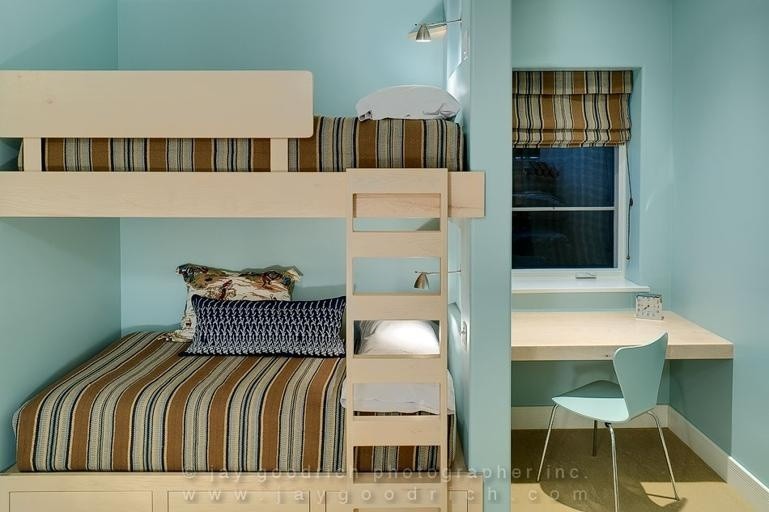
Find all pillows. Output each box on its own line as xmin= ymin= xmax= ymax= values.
xmin=335 ymin=314 xmax=456 ymax=418
xmin=156 ymin=256 xmax=345 ymax=359
xmin=354 ymin=82 xmax=462 ymax=122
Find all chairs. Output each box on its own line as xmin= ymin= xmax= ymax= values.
xmin=535 ymin=330 xmax=682 ymax=512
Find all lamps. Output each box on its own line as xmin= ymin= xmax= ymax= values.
xmin=413 ymin=16 xmax=462 ymax=43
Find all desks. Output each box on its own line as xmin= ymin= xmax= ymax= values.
xmin=509 ymin=307 xmax=733 ymax=363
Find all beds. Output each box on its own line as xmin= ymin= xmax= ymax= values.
xmin=0 ymin=68 xmax=486 ymax=512
